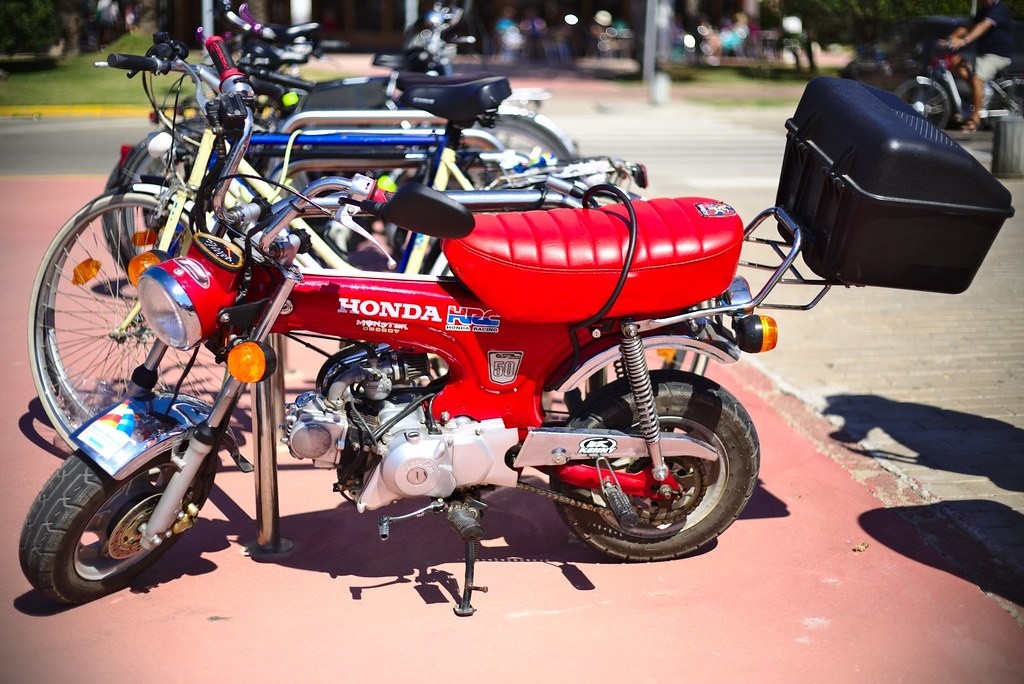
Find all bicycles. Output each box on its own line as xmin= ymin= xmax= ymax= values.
xmin=896 ymin=38 xmax=1024 ymax=138
xmin=27 ymin=0 xmax=650 ymax=452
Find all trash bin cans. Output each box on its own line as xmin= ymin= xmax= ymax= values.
xmin=991 ymin=114 xmax=1023 ymax=182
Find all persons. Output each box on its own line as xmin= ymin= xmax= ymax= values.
xmin=856 ymin=42 xmax=885 ymax=63
xmin=82 ymin=0 xmax=137 ymax=45
xmin=939 ymin=0 xmax=1015 ymax=130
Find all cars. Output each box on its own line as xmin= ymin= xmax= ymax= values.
xmin=842 ymin=14 xmax=1017 ymax=122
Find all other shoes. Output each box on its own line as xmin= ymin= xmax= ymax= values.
xmin=962 ymin=120 xmax=979 ymax=132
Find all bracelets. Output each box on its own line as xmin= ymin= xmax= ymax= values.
xmin=962 ymin=37 xmax=969 ymax=44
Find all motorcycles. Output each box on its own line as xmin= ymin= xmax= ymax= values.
xmin=19 ymin=34 xmax=1016 ymax=618
